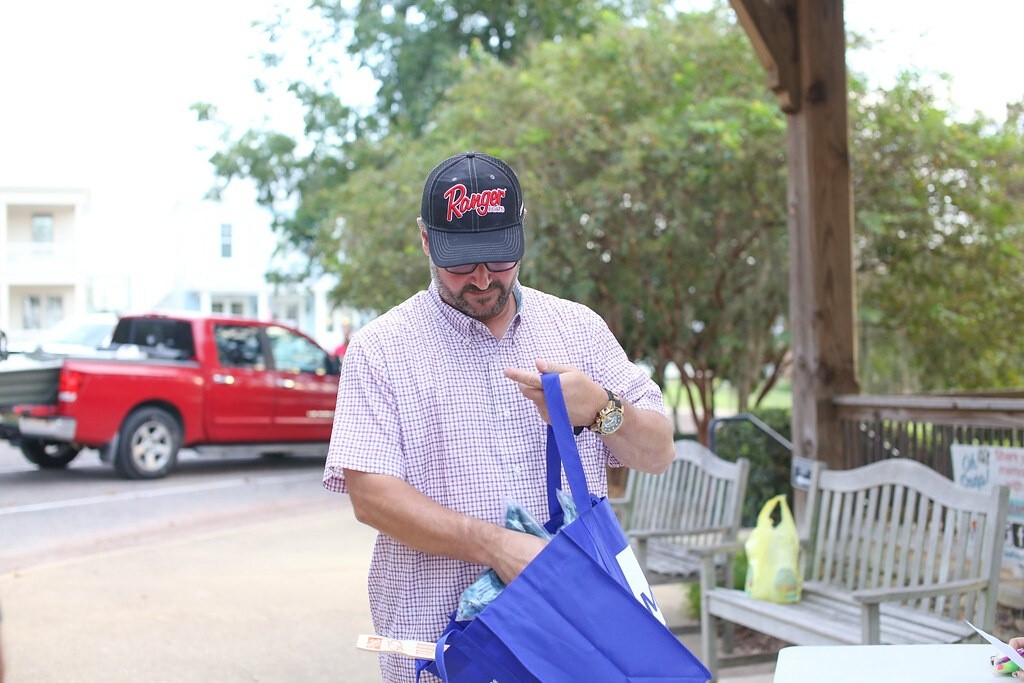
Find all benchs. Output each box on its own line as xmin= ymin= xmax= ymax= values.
xmin=676 ymin=455 xmax=1011 ymax=683
xmin=609 ymin=440 xmax=751 ymax=653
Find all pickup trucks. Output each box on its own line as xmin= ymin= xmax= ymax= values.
xmin=0 ymin=314 xmax=348 ymax=479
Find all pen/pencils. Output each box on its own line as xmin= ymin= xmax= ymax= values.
xmin=992 ymin=648 xmax=1023 ymax=674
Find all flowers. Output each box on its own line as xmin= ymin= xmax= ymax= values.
xmin=990 ymin=648 xmax=1024 ymax=679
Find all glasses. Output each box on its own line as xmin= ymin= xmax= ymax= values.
xmin=445 ymin=261 xmax=517 ymax=274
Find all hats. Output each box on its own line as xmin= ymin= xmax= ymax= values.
xmin=420 ymin=152 xmax=524 ymax=267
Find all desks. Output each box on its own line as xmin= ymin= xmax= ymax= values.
xmin=773 ymin=644 xmax=1024 ymax=683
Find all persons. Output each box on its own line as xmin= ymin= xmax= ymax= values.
xmin=321 ymin=151 xmax=676 ymax=683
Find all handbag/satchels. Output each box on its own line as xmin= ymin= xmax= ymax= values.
xmin=744 ymin=494 xmax=802 ymax=603
xmin=415 ymin=374 xmax=711 ymax=683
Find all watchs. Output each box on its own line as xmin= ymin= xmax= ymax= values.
xmin=573 ymin=388 xmax=625 ymax=437
